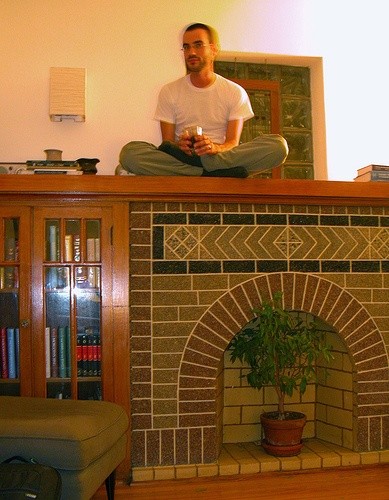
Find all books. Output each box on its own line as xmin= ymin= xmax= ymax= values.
xmin=353 ymin=165 xmax=389 ymax=182
xmin=45 ymin=327 xmax=102 ymax=378
xmin=48 ymin=225 xmax=101 ymax=289
xmin=0 ymin=327 xmax=20 ymax=379
xmin=0 ymin=236 xmax=19 ymax=289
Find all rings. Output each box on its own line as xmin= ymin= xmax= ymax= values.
xmin=208 ymin=145 xmax=212 ymax=149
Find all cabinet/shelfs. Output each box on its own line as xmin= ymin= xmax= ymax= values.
xmin=0 ymin=200 xmax=133 ymax=480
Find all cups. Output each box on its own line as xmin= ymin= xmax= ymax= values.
xmin=76 ymin=158 xmax=100 ymax=175
xmin=45 ymin=149 xmax=62 ymax=161
xmin=185 ymin=126 xmax=202 ymax=153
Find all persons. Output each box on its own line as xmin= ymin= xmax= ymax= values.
xmin=119 ymin=23 xmax=289 ymax=178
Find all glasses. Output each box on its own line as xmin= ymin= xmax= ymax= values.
xmin=180 ymin=43 xmax=216 ymax=51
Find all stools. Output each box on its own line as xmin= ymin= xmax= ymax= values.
xmin=0 ymin=396 xmax=131 ymax=500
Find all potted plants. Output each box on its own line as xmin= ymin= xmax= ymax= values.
xmin=227 ymin=291 xmax=336 ymax=457
